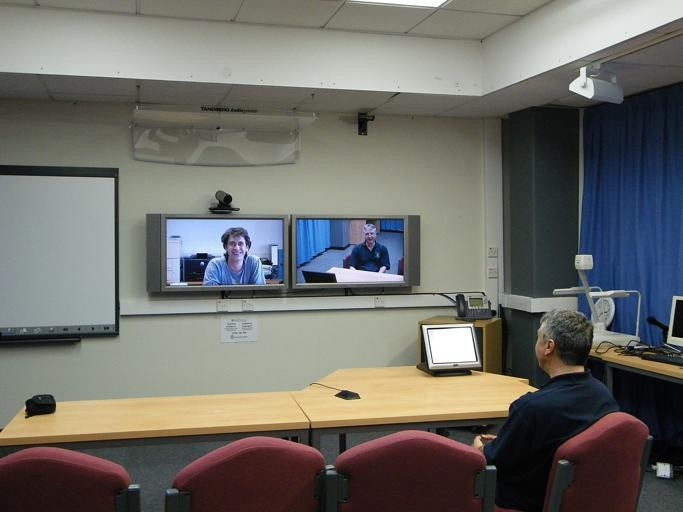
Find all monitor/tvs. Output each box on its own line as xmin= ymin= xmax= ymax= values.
xmin=146 ymin=212 xmax=290 ymax=293
xmin=417 ymin=322 xmax=483 ymax=378
xmin=667 ymin=295 xmax=683 ymax=347
xmin=291 ymin=213 xmax=421 ymax=290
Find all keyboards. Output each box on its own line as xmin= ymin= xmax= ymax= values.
xmin=643 ymin=354 xmax=683 ymax=367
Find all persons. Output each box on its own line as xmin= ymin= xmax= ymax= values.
xmin=203 ymin=227 xmax=267 ymax=285
xmin=472 ymin=308 xmax=621 ymax=511
xmin=346 ymin=224 xmax=390 ymax=273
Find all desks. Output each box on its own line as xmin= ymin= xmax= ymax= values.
xmin=589 ymin=334 xmax=683 ymax=471
xmin=0 ymin=392 xmax=312 ymax=447
xmin=292 ymin=366 xmax=539 ymax=457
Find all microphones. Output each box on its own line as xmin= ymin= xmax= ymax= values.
xmin=646 ymin=315 xmax=668 ymax=331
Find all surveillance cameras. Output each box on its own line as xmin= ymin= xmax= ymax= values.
xmin=209 ymin=190 xmax=241 ymax=217
xmin=356 ymin=110 xmax=376 ymax=137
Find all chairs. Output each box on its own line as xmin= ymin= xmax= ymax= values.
xmin=540 ymin=413 xmax=653 ymax=511
xmin=164 ymin=436 xmax=333 ymax=512
xmin=0 ymin=444 xmax=140 ymax=512
xmin=333 ymin=430 xmax=498 ymax=512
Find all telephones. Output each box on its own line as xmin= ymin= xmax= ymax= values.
xmin=454 ymin=289 xmax=494 ymax=321
